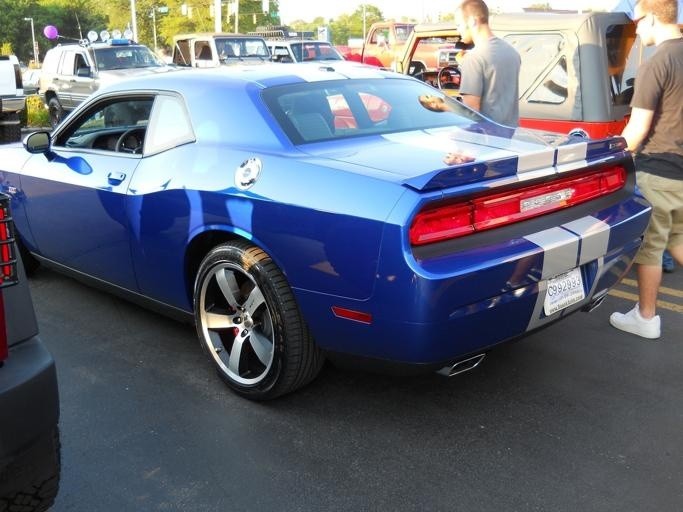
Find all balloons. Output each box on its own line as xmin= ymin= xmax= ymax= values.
xmin=42 ymin=25 xmax=58 ymax=41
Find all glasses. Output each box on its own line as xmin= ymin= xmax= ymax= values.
xmin=631 ymin=14 xmax=649 ymax=29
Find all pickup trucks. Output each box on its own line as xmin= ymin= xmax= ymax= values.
xmin=341 ymin=19 xmax=412 ymax=63
xmin=0 ymin=56 xmax=26 ymax=144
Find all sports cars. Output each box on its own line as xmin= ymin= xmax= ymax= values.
xmin=0 ymin=61 xmax=652 ymax=398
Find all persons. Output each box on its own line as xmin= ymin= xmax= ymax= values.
xmin=608 ymin=0 xmax=683 ymax=338
xmin=455 ymin=1 xmax=522 ymax=129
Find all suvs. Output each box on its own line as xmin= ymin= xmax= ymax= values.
xmin=37 ymin=40 xmax=185 ymax=130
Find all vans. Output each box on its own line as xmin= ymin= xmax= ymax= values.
xmin=382 ymin=12 xmax=636 ymax=126
xmin=264 ymin=41 xmax=345 ymax=63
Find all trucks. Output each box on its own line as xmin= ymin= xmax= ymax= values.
xmin=172 ymin=34 xmax=271 ymax=71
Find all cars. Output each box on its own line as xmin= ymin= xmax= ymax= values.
xmin=0 ymin=199 xmax=60 ymax=512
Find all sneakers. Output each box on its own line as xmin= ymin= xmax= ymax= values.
xmin=609 ymin=301 xmax=661 ymax=339
